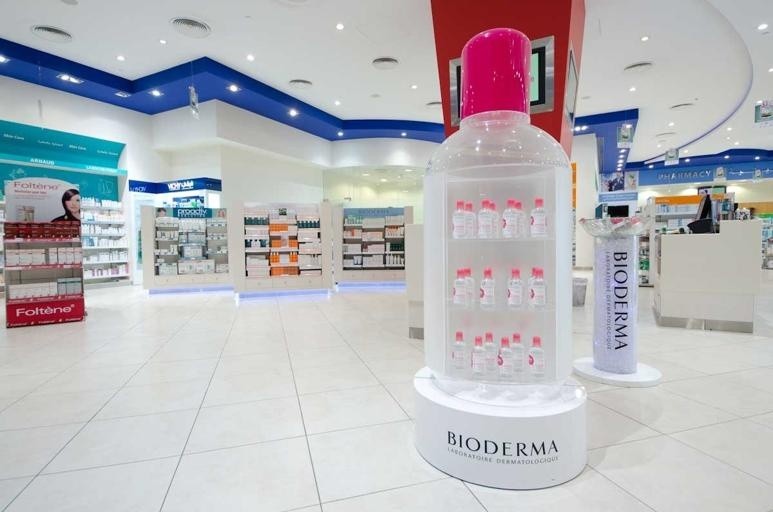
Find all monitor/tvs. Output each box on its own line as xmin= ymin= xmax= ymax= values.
xmin=695 ymin=195 xmax=712 ymax=220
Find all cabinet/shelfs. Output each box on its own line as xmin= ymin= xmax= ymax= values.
xmin=1 ymin=152 xmax=130 ymax=299
xmin=635 ymin=193 xmax=773 ymax=287
xmin=4 ymin=221 xmax=85 ymax=328
xmin=423 ymin=109 xmax=573 ymax=409
xmin=141 ymin=203 xmax=413 ymax=294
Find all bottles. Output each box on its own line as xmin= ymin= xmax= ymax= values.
xmin=736 ymin=207 xmax=751 ymax=220
xmin=289 ymin=251 xmax=298 ymax=263
xmin=389 ymin=223 xmax=402 ymax=251
xmin=452 ymin=328 xmax=545 ymax=381
xmin=579 ymin=216 xmax=646 ymax=237
xmin=81 ymin=224 xmax=125 ymax=236
xmin=270 ymin=252 xmax=279 ymax=263
xmin=246 ymin=216 xmax=267 ymax=224
xmin=81 ymin=196 xmax=123 ymax=209
xmin=452 ymin=199 xmax=546 ymax=239
xmin=455 ymin=264 xmax=546 ymax=312
xmin=97 ymin=213 xmax=123 ymax=222
xmin=299 ymin=219 xmax=320 ymax=229
xmin=722 ymin=198 xmax=731 ymax=211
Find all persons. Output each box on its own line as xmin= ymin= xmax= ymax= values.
xmin=157 ymin=208 xmax=166 ymax=217
xmin=49 ymin=188 xmax=80 ymax=221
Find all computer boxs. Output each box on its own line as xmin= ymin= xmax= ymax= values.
xmin=687 ymin=219 xmax=714 ymax=233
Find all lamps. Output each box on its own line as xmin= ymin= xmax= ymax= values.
xmin=169 ymin=16 xmax=211 ymax=121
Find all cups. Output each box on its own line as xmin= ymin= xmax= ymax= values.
xmin=722 ymin=213 xmax=727 ymax=221
